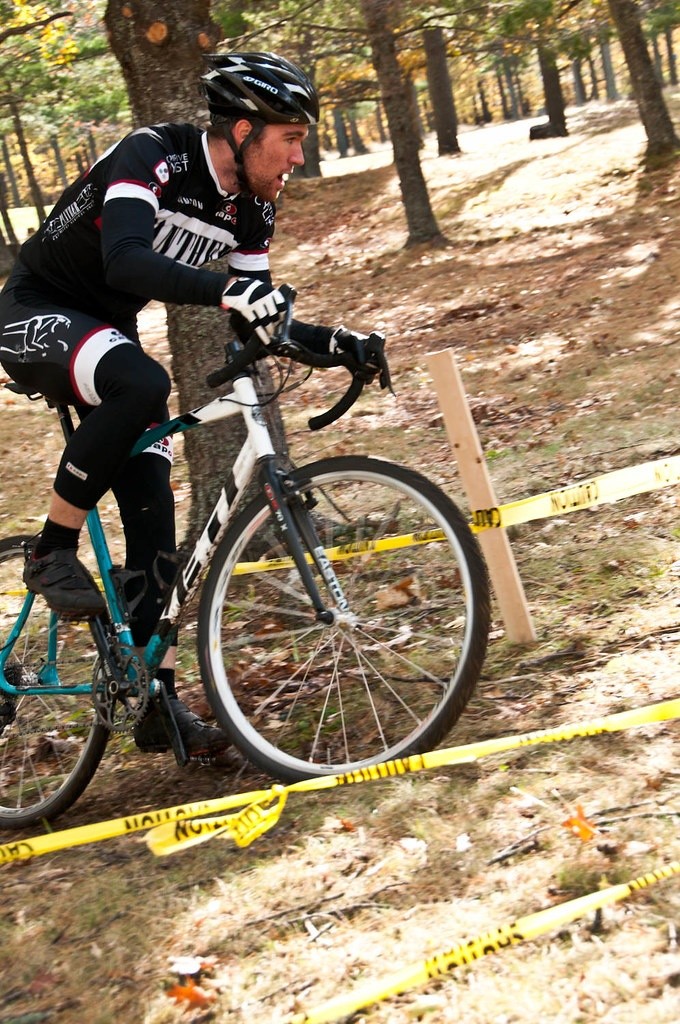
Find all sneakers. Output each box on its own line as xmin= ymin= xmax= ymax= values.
xmin=134 ymin=699 xmax=233 ymax=756
xmin=23 ymin=547 xmax=106 ymax=618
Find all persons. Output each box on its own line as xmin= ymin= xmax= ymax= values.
xmin=0 ymin=49 xmax=383 ymax=752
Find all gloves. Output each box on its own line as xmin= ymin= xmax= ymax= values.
xmin=329 ymin=324 xmax=391 ymax=390
xmin=218 ymin=277 xmax=288 ymax=344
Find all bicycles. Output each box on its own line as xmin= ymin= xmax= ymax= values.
xmin=0 ymin=282 xmax=490 ymax=831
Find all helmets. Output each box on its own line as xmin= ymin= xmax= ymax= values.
xmin=200 ymin=51 xmax=318 ymax=124
